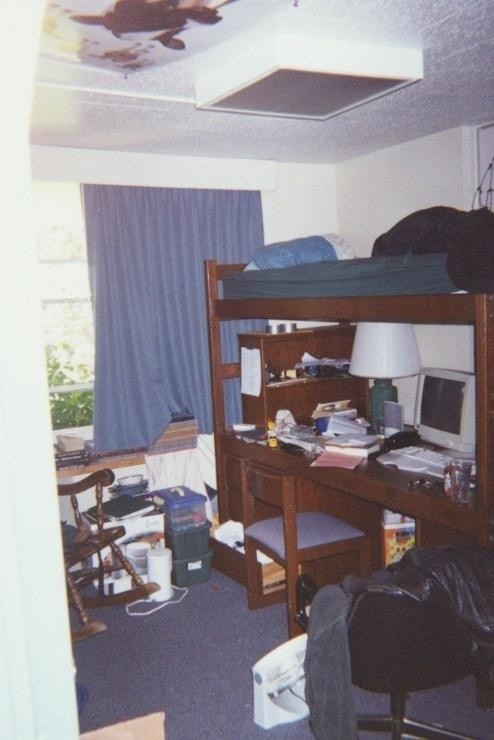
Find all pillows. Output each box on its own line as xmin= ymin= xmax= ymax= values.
xmin=250 ymin=233 xmax=355 ymax=269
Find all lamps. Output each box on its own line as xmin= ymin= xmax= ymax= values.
xmin=347 ymin=322 xmax=423 ymax=429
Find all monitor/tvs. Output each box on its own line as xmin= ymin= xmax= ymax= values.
xmin=413 ymin=367 xmax=476 ymax=459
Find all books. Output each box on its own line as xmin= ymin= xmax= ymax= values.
xmin=311 ymin=399 xmax=380 ymax=458
xmin=52 ymin=410 xmax=198 ymax=477
xmin=87 ymin=495 xmax=155 ymax=523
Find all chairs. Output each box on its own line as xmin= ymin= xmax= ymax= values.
xmin=297 ymin=545 xmax=493 ymax=740
xmin=57 ymin=468 xmax=160 ymax=642
xmin=236 ymin=456 xmax=372 ymax=640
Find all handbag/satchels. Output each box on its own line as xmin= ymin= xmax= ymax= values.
xmin=339 ymin=557 xmax=480 ymax=692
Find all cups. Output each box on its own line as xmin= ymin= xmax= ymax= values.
xmin=450 ymin=461 xmax=472 ymax=504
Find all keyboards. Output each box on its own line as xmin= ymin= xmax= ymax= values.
xmin=377 ymin=445 xmax=476 ymax=478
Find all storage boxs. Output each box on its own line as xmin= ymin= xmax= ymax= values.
xmin=78 ymin=486 xmax=212 ymax=594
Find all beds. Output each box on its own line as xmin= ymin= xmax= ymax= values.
xmin=203 ymin=253 xmax=494 ymax=325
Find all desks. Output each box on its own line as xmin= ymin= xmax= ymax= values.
xmin=226 ymin=325 xmax=476 ymax=588
